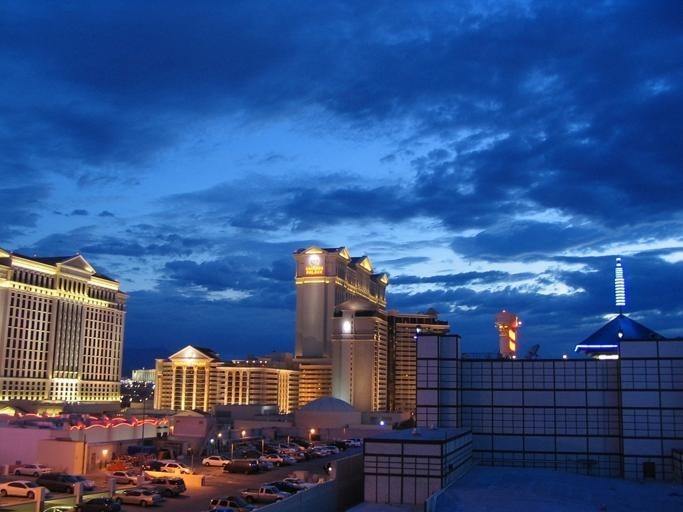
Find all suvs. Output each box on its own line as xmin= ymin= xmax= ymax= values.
xmin=142 ymin=476 xmax=186 ymax=496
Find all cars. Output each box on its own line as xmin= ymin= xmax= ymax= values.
xmin=76 ymin=498 xmax=121 ymax=512
xmin=0 ymin=480 xmax=50 ymax=499
xmin=112 ymin=488 xmax=161 ymax=507
xmin=112 ymin=470 xmax=138 ymax=484
xmin=140 ymin=485 xmax=160 ymax=493
xmin=13 ymin=463 xmax=52 ymax=477
xmin=200 ymin=438 xmax=363 ymax=475
xmin=35 ymin=472 xmax=95 ymax=494
xmin=159 ymin=463 xmax=191 ymax=475
xmin=42 ymin=505 xmax=75 ymax=512
xmin=208 ymin=477 xmax=316 ymax=511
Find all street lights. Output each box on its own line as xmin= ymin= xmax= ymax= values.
xmin=133 ymin=380 xmax=156 ymax=440
xmin=309 ymin=429 xmax=315 ymax=441
